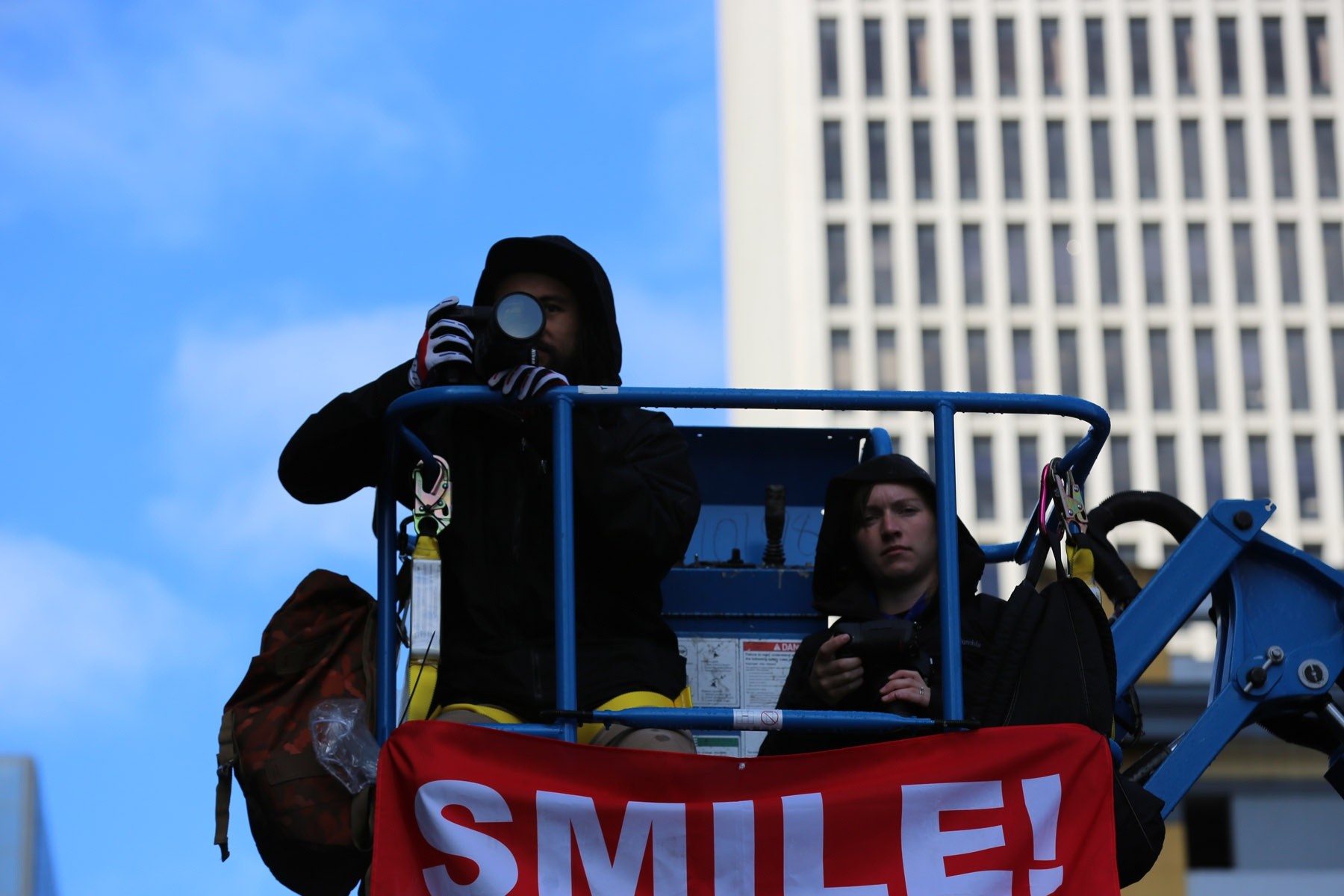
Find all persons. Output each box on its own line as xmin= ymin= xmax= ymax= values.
xmin=275 ymin=236 xmax=702 ymax=761
xmin=757 ymin=453 xmax=1033 ymax=761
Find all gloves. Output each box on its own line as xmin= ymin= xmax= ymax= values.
xmin=488 ymin=362 xmax=572 ymax=406
xmin=409 ymin=296 xmax=476 ymax=389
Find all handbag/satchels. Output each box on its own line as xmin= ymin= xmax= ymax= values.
xmin=1007 ymin=529 xmax=1165 ymax=889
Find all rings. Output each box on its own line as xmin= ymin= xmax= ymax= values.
xmin=919 ymin=686 xmax=924 ymax=695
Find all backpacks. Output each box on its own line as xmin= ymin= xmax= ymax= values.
xmin=214 ymin=569 xmax=410 ymax=896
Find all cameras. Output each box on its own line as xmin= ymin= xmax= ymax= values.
xmin=444 ymin=290 xmax=545 ymax=375
xmin=830 ymin=617 xmax=935 ymax=688
